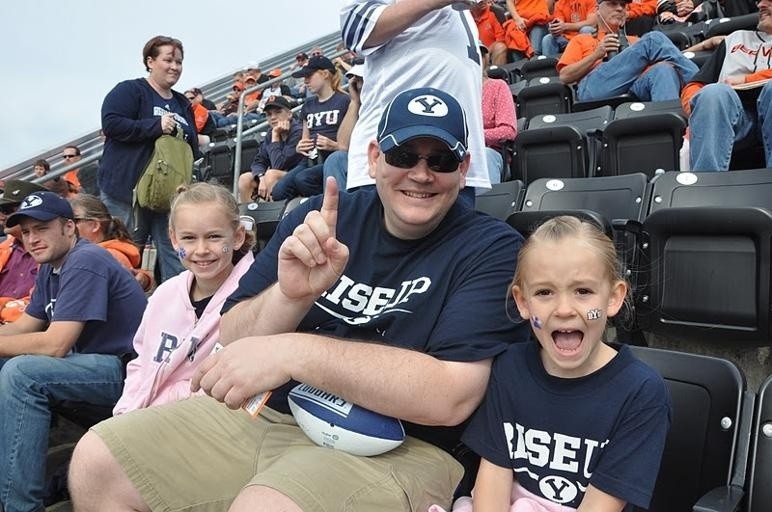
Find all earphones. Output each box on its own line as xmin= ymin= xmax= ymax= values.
xmin=596 ymin=9 xmax=600 ymax=15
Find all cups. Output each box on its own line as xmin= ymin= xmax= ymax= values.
xmin=451 ymin=2 xmax=467 ymax=12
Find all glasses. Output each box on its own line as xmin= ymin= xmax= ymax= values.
xmin=384 ymin=149 xmax=460 ymax=173
xmin=184 ymin=96 xmax=199 ymax=101
xmin=61 ymin=153 xmax=81 ymax=160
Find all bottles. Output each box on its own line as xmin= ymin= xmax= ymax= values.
xmin=680 ymin=136 xmax=689 ymax=171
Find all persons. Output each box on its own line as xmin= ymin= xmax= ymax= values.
xmin=680 ymin=0 xmax=772 ymax=172
xmin=450 ymin=215 xmax=673 ymax=512
xmin=182 ymin=42 xmax=364 ymax=204
xmin=0 ymin=177 xmax=153 ymax=363
xmin=473 ymin=0 xmax=727 ymax=183
xmin=109 ymin=183 xmax=256 ymax=417
xmin=70 ymin=87 xmax=525 ymax=512
xmin=35 ymin=145 xmax=101 ymax=197
xmin=339 ymin=0 xmax=491 ymax=206
xmin=0 ymin=190 xmax=148 ymax=512
xmin=97 ymin=36 xmax=200 ymax=284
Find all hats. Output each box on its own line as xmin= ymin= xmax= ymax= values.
xmin=232 ymin=50 xmax=368 ymax=114
xmin=1 ymin=178 xmax=80 ymax=230
xmin=376 ymin=86 xmax=469 ymax=165
xmin=472 ymin=40 xmax=493 ymax=57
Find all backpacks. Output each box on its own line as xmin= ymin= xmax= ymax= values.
xmin=131 ymin=126 xmax=195 ymax=213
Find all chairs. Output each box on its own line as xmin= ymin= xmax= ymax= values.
xmin=21 ymin=1 xmax=772 ymax=512
